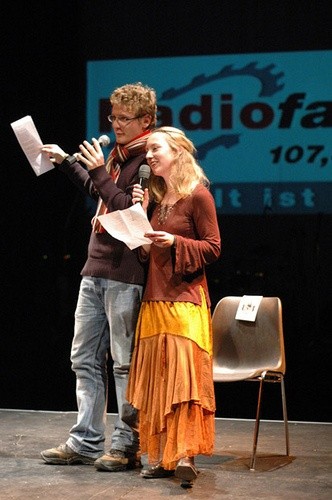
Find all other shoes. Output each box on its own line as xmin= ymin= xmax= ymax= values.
xmin=140 ymin=464 xmax=176 ymax=478
xmin=175 ymin=460 xmax=198 ymax=480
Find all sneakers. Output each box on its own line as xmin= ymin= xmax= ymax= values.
xmin=94 ymin=448 xmax=137 ymax=471
xmin=40 ymin=443 xmax=98 ymax=464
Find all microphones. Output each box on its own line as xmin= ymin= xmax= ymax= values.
xmin=66 ymin=135 xmax=110 ymax=166
xmin=136 ymin=165 xmax=151 ymax=206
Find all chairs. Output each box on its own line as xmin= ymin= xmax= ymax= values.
xmin=212 ymin=296 xmax=293 ymax=469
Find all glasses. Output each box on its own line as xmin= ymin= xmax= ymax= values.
xmin=108 ymin=113 xmax=146 ymax=123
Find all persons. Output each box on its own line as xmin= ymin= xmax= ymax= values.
xmin=126 ymin=127 xmax=221 ymax=488
xmin=40 ymin=82 xmax=155 ymax=470
